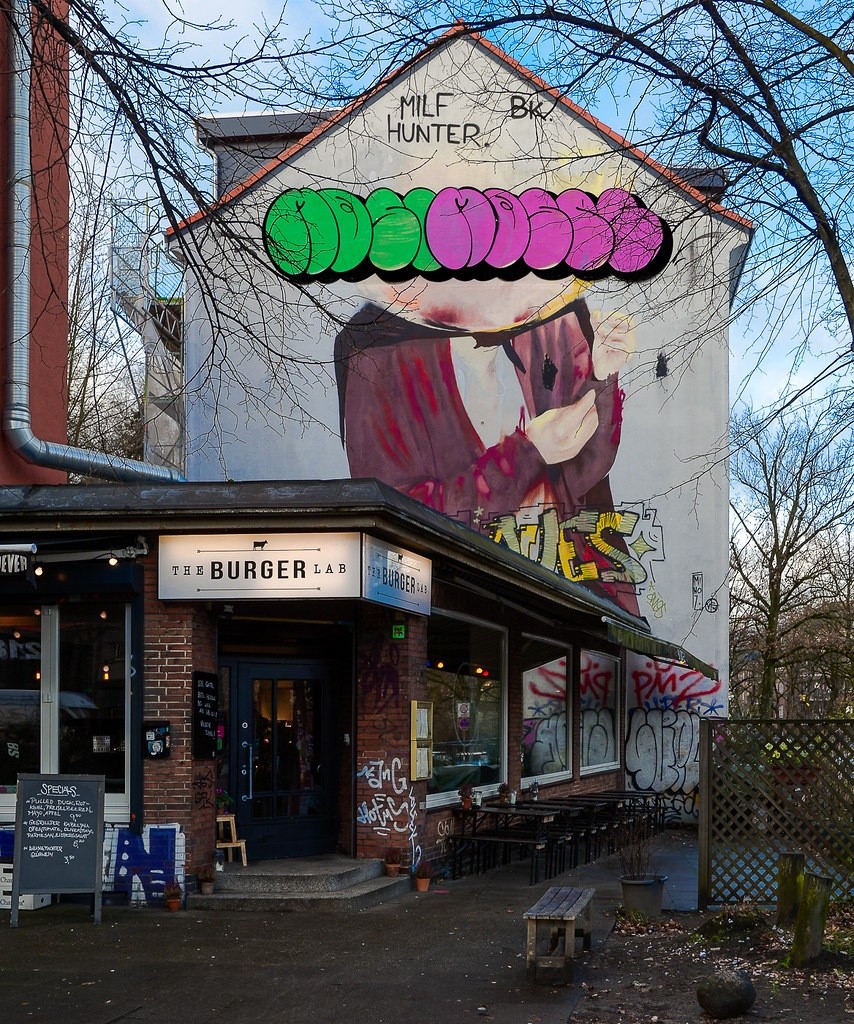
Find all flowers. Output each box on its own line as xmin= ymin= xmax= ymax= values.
xmin=458 ymin=783 xmax=476 ymax=800
xmin=528 ymin=779 xmax=543 ymax=797
xmin=497 ymin=781 xmax=516 ymax=795
xmin=214 ymin=850 xmax=224 ymax=866
xmin=162 ymin=875 xmax=184 ymax=900
xmin=215 ymin=786 xmax=235 ymax=808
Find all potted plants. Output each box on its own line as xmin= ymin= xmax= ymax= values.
xmin=604 ymin=798 xmax=669 ymax=925
xmin=414 ymin=859 xmax=437 ymax=891
xmin=383 ymin=847 xmax=400 ymax=877
xmin=194 ymin=865 xmax=215 ymax=894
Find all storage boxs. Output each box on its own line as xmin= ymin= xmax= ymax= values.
xmin=0 ymin=863 xmax=13 ymax=886
xmin=0 ymin=886 xmax=51 ymax=910
xmin=0 ymin=825 xmax=14 ymax=863
xmin=0 ymin=794 xmax=18 ymax=822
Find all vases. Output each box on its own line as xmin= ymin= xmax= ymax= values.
xmin=461 ymin=797 xmax=473 ymax=809
xmin=500 ymin=793 xmax=510 ymax=803
xmin=533 ymin=788 xmax=538 ymax=801
xmin=216 ymin=809 xmax=225 ymax=815
xmin=215 ymin=861 xmax=225 ymax=872
xmin=167 ymin=899 xmax=180 ymax=912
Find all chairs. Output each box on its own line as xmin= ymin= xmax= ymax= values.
xmin=215 ymin=815 xmax=247 ymax=865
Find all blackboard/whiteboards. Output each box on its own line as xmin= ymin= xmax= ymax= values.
xmin=192 ymin=671 xmax=219 ymax=761
xmin=13 ymin=773 xmax=105 ymax=893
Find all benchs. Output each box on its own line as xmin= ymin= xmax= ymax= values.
xmin=448 ymin=806 xmax=671 ymax=887
xmin=522 ymin=887 xmax=596 ymax=967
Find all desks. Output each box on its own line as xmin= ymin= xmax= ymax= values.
xmin=451 ymin=790 xmax=664 ymax=884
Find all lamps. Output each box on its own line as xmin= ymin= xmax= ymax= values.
xmin=35 ymin=558 xmax=44 ymax=576
xmin=87 ymin=552 xmax=120 ymax=566
xmin=475 ymin=662 xmax=482 ymax=675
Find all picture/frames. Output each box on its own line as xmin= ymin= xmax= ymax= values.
xmin=115 ymin=641 xmax=125 ymax=661
